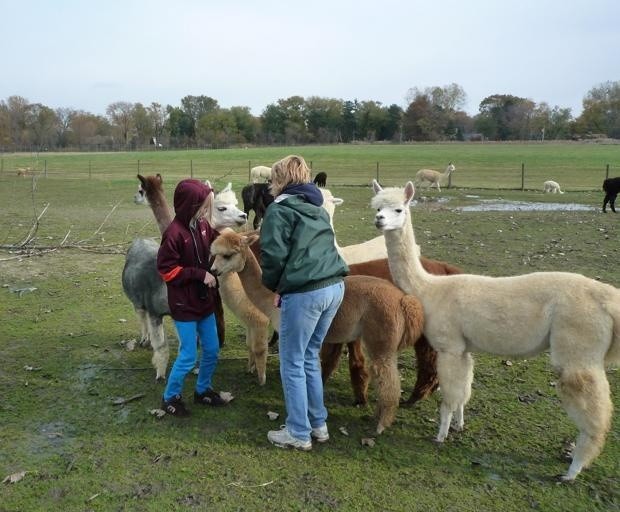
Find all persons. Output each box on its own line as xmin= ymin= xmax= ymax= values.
xmin=158 ymin=180 xmax=227 ymax=417
xmin=258 ymin=154 xmax=350 ymax=450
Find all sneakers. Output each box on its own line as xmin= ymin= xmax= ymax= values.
xmin=311 ymin=423 xmax=330 ymax=444
xmin=267 ymin=426 xmax=313 ymax=452
xmin=194 ymin=387 xmax=226 ymax=407
xmin=161 ymin=394 xmax=192 ymax=418
